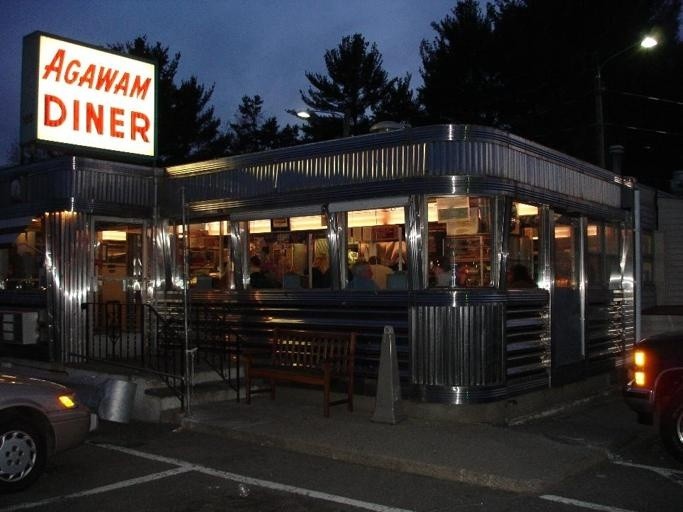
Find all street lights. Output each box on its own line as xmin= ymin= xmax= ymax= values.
xmin=595 ymin=34 xmax=659 ymax=168
xmin=298 ymin=108 xmax=354 ymax=140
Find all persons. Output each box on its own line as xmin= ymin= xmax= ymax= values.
xmin=154 ymin=245 xmax=579 ymax=293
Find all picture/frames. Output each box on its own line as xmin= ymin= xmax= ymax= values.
xmin=271 ymin=217 xmax=289 ymax=231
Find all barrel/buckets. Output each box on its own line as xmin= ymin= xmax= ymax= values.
xmin=98 ymin=379 xmax=137 ymax=424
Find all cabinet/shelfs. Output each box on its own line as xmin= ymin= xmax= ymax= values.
xmin=442 ymin=235 xmax=534 ymax=287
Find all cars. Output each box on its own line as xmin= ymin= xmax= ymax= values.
xmin=626 ymin=332 xmax=683 ymax=457
xmin=0 ymin=374 xmax=90 ymax=491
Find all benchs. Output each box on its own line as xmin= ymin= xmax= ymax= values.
xmin=245 ymin=329 xmax=356 ymax=417
